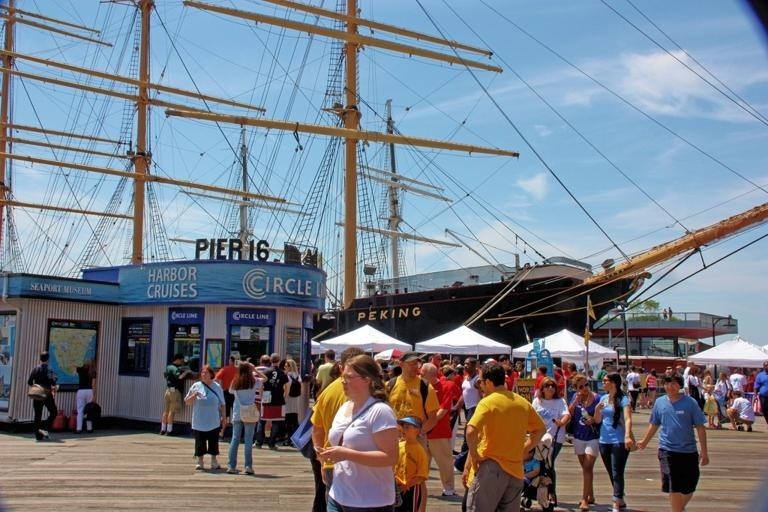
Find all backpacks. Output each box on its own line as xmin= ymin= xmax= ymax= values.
xmin=289 ymin=375 xmax=301 ymax=397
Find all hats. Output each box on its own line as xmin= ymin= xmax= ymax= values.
xmin=396 ymin=417 xmax=421 ymax=429
xmin=400 ymin=352 xmax=426 ymax=363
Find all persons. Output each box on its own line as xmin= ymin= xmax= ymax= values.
xmin=308 ymin=350 xmax=710 ymax=511
xmin=70 ymin=357 xmax=102 ymax=433
xmin=25 ymin=349 xmax=57 ymax=441
xmin=184 ymin=353 xmax=302 ymax=474
xmin=685 ymin=360 xmax=768 ymax=431
xmin=160 ymin=353 xmax=193 ymax=436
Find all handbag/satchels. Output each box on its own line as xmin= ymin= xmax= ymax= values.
xmin=633 ymin=382 xmax=641 ymax=388
xmin=290 ymin=407 xmax=321 ymax=458
xmin=261 ymin=390 xmax=272 ymax=404
xmin=28 ymin=383 xmax=48 ymax=401
xmin=239 ymin=403 xmax=261 ymax=423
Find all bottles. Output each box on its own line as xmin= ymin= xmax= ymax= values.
xmin=580 ymin=408 xmax=589 ymax=419
xmin=191 ymin=327 xmax=199 ymax=333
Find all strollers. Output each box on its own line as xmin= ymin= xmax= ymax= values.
xmin=521 ymin=420 xmax=561 ymax=512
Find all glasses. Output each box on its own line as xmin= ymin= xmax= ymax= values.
xmin=602 ymin=378 xmax=609 ymax=384
xmin=579 ymin=383 xmax=589 ymax=389
xmin=543 ymin=383 xmax=555 ymax=388
xmin=341 ymin=373 xmax=360 ymax=382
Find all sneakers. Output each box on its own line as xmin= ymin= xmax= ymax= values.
xmin=211 ymin=460 xmax=220 ymax=469
xmin=196 ymin=461 xmax=204 ymax=470
xmin=165 ymin=431 xmax=176 ymax=435
xmin=38 ymin=429 xmax=50 ymax=438
xmin=159 ymin=430 xmax=166 ymax=435
xmin=442 ymin=489 xmax=464 ymax=502
xmin=245 ymin=466 xmax=254 ymax=473
xmin=707 ymin=423 xmax=752 ymax=432
xmin=226 ymin=467 xmax=239 ymax=473
xmin=252 ymin=443 xmax=261 ymax=449
xmin=607 ymin=504 xmax=627 ymax=510
